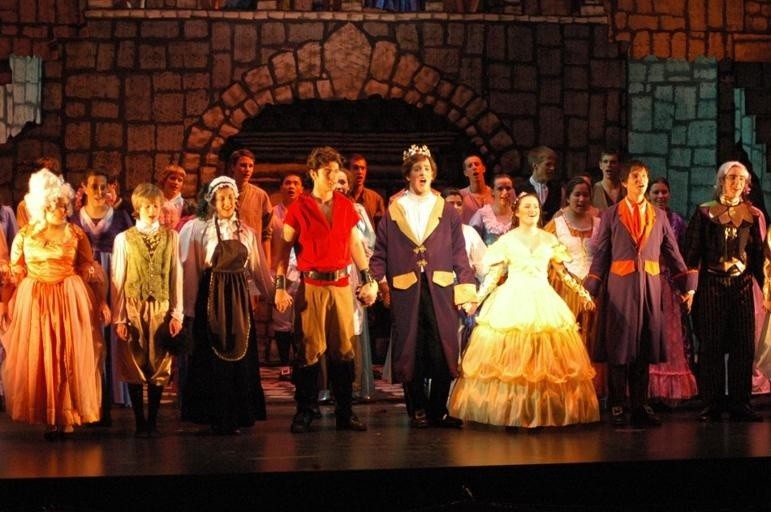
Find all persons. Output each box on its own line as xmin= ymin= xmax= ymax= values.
xmin=3 ymin=169 xmax=111 ymax=435
xmin=178 ymin=176 xmax=295 ymax=431
xmin=20 ymin=143 xmax=770 ymax=410
xmin=356 ymin=143 xmax=477 ymax=427
xmin=577 ymin=156 xmax=698 ymax=430
xmin=110 ymin=182 xmax=183 ymax=439
xmin=442 ymin=191 xmax=599 ymax=429
xmin=682 ymin=159 xmax=767 ymax=425
xmin=272 ymin=146 xmax=379 ymax=431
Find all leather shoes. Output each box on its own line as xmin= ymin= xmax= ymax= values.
xmin=608 ymin=404 xmax=658 ymax=425
xmin=410 ymin=407 xmax=463 ymax=427
xmin=698 ymin=399 xmax=764 ymax=422
xmin=291 ymin=409 xmax=366 ymax=433
xmin=45 ymin=424 xmax=75 ymax=440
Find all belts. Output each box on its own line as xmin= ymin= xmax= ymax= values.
xmin=300 ymin=269 xmax=347 ymax=280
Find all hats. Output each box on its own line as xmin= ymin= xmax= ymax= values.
xmin=207 ymin=176 xmax=239 ymax=199
